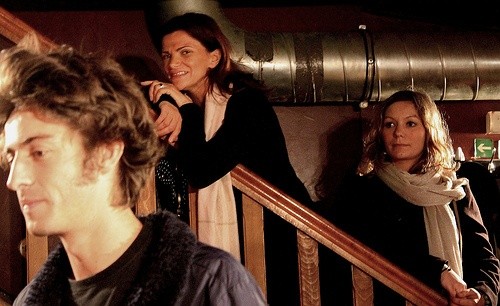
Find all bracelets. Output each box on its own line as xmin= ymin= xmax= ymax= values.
xmin=442 ymin=266 xmax=452 ymax=273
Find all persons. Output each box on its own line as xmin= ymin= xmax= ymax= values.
xmin=0 ymin=46 xmax=267 ymax=306
xmin=317 ymin=90 xmax=500 ymax=306
xmin=117 ymin=11 xmax=328 ymax=305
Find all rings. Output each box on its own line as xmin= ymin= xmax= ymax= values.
xmin=158 ymin=83 xmax=165 ymax=89
